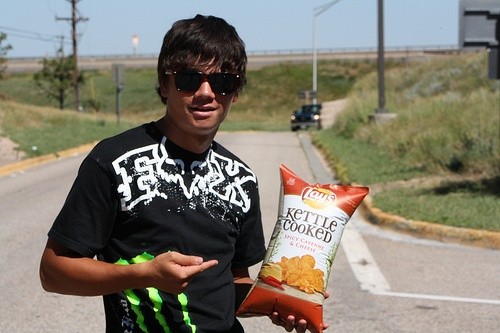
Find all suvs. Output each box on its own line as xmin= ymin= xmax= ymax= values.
xmin=290 ymin=103 xmax=323 ymax=131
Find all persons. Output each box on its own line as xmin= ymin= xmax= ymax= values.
xmin=39 ymin=14 xmax=329 ymax=333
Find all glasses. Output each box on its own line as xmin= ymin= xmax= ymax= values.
xmin=165 ymin=67 xmax=241 ymax=96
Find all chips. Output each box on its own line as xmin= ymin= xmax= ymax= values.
xmin=281 ymin=256 xmax=325 ymax=294
